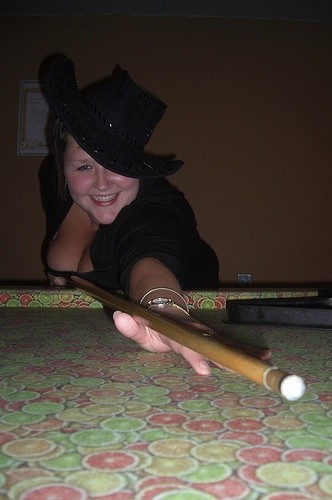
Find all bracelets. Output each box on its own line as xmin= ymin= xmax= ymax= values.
xmin=137 ymin=286 xmax=190 ymax=313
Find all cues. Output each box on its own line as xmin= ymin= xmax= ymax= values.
xmin=66 ymin=276 xmax=305 ymax=403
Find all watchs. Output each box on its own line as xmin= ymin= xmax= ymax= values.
xmin=143 ymin=296 xmax=190 ymax=317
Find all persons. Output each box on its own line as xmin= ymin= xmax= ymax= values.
xmin=44 ymin=65 xmax=274 ymax=377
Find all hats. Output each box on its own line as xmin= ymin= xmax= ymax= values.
xmin=38 ymin=52 xmax=177 ymax=178
